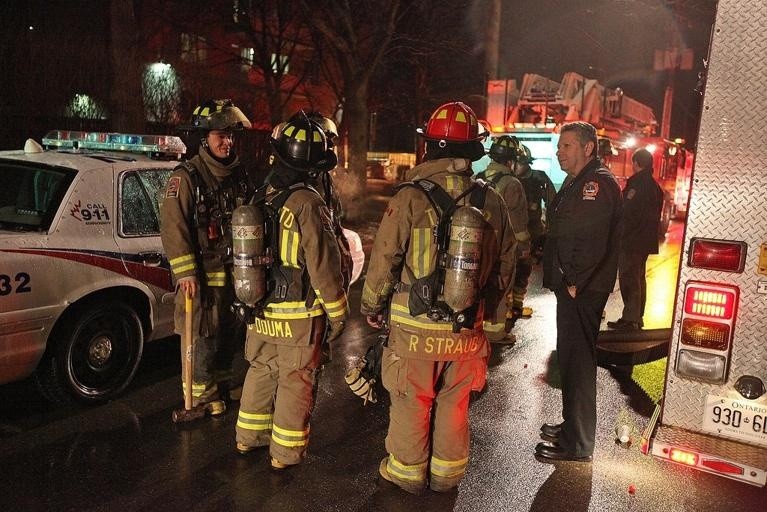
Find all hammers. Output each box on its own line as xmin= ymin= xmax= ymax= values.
xmin=172 ymin=290 xmax=204 ymax=422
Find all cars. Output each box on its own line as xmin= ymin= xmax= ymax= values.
xmin=0 ymin=128 xmax=367 ymax=408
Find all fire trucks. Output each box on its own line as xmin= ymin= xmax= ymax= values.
xmin=634 ymin=1 xmax=767 ymax=490
xmin=473 ymin=73 xmax=686 ymax=239
xmin=671 ymin=148 xmax=693 ymax=221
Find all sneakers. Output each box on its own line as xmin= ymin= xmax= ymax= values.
xmin=203 ymin=398 xmax=229 ymax=414
xmin=237 ymin=442 xmax=288 ymax=469
xmin=522 ymin=307 xmax=533 ymax=317
xmin=379 ymin=456 xmax=393 ymax=481
xmin=489 ymin=334 xmax=516 ymax=345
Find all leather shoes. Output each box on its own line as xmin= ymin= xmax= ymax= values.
xmin=606 ymin=321 xmax=637 ymax=328
xmin=536 ymin=443 xmax=592 ymax=460
xmin=541 ymin=423 xmax=565 ymax=437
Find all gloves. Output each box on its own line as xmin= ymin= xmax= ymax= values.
xmin=326 ymin=323 xmax=345 ymax=345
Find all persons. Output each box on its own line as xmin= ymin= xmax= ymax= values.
xmin=477 ymin=136 xmax=530 ymax=346
xmin=534 ymin=121 xmax=623 ymax=461
xmin=361 ymin=101 xmax=516 ymax=493
xmin=505 ymin=144 xmax=556 ymax=318
xmin=605 ymin=148 xmax=666 ymax=330
xmin=266 ymin=108 xmax=354 ymax=366
xmin=227 ymin=126 xmax=349 ymax=473
xmin=156 ymin=99 xmax=251 ymax=417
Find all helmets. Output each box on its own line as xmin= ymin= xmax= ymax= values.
xmin=272 ymin=111 xmax=337 ymax=174
xmin=417 ymin=102 xmax=492 ymax=143
xmin=488 ymin=135 xmax=535 ymax=164
xmin=190 ymin=99 xmax=251 ymax=132
